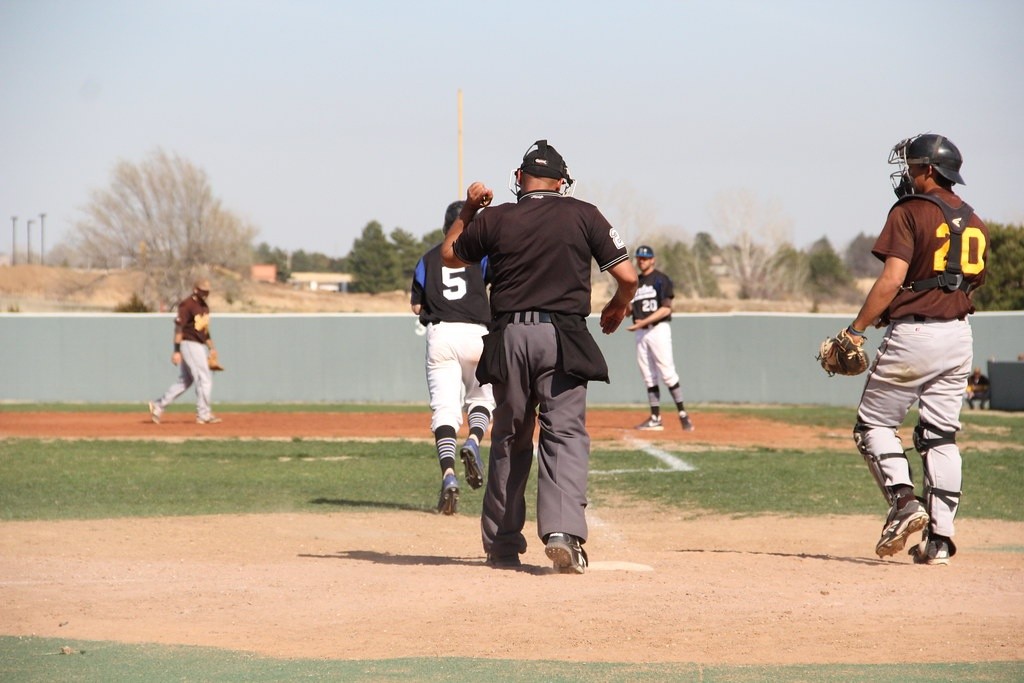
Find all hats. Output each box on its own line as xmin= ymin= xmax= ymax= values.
xmin=521 ymin=149 xmax=566 ymax=179
xmin=634 ymin=245 xmax=653 ymax=257
xmin=194 ymin=277 xmax=214 ymax=291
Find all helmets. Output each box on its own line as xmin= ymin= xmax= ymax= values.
xmin=442 ymin=201 xmax=478 ymax=233
xmin=906 ymin=134 xmax=967 ymax=186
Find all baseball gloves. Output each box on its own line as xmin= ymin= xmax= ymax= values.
xmin=821 ymin=325 xmax=870 ymax=377
xmin=208 ymin=354 xmax=225 ymax=371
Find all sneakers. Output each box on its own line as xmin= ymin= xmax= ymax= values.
xmin=487 ymin=552 xmax=521 ymax=566
xmin=545 ymin=531 xmax=588 ymax=574
xmin=875 ymin=499 xmax=929 ymax=557
xmin=438 ymin=473 xmax=460 ymax=516
xmin=460 ymin=438 xmax=485 ymax=490
xmin=908 ymin=539 xmax=951 ymax=565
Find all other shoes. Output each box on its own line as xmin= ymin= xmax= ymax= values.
xmin=195 ymin=415 xmax=222 ymax=424
xmin=634 ymin=415 xmax=664 ymax=430
xmin=147 ymin=400 xmax=162 ymax=424
xmin=678 ymin=410 xmax=694 ymax=432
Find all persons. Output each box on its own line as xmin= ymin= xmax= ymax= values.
xmin=966 ymin=367 xmax=991 ymax=410
xmin=148 ymin=279 xmax=222 ymax=425
xmin=814 ymin=134 xmax=991 ymax=566
xmin=410 ymin=201 xmax=496 ymax=515
xmin=622 ymin=245 xmax=695 ymax=433
xmin=437 ymin=140 xmax=638 ymax=576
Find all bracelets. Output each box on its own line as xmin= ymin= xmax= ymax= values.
xmin=175 ymin=343 xmax=180 ymax=353
xmin=850 ymin=322 xmax=865 ymax=336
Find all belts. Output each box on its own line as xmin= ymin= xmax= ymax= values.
xmin=508 ymin=312 xmax=552 ymax=323
